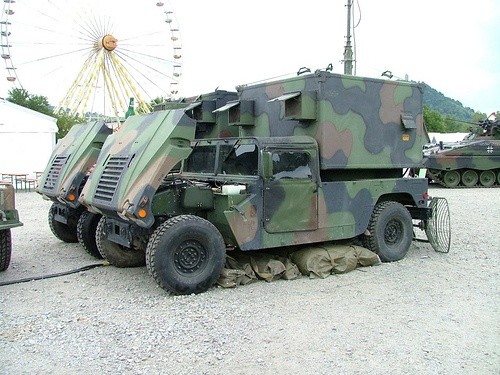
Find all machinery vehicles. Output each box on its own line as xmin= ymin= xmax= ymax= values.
xmin=0 ymin=64 xmax=500 ymax=297
